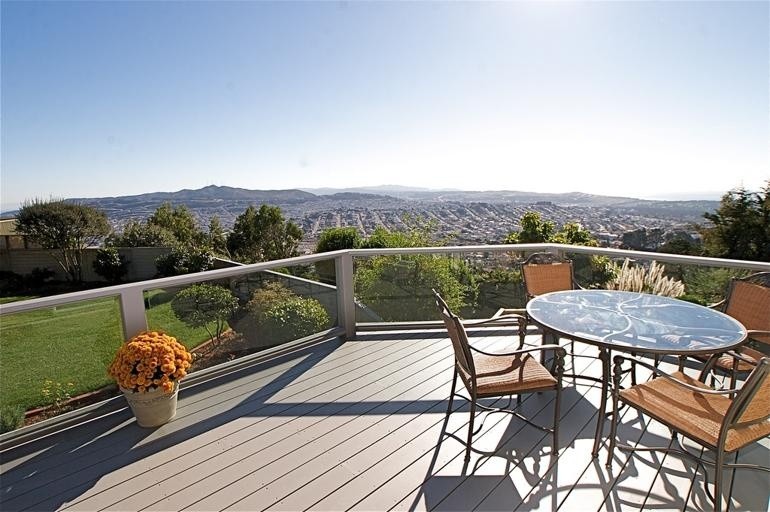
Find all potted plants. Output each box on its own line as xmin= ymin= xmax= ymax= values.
xmin=105 ymin=329 xmax=195 ymax=429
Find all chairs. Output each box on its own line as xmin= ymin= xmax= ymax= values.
xmin=427 ymin=287 xmax=567 ymax=464
xmin=605 ymin=330 xmax=770 ymax=512
xmin=671 ymin=270 xmax=770 ymax=405
xmin=518 ymin=252 xmax=588 ymax=387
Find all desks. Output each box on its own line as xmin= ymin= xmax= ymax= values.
xmin=520 ymin=287 xmax=749 ymax=459
